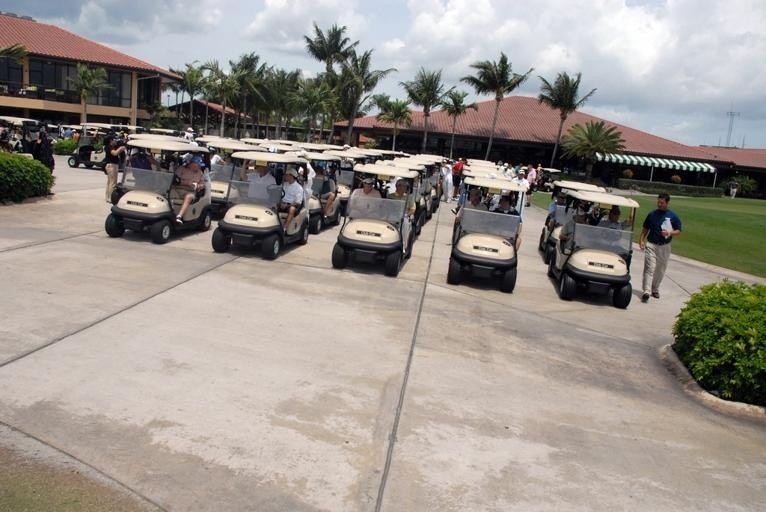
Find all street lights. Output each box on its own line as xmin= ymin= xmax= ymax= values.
xmin=14 ymin=88 xmax=27 ymax=115
xmin=134 ymin=73 xmax=160 ymax=127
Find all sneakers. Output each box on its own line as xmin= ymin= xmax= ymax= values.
xmin=643 ymin=293 xmax=649 ymax=301
xmin=451 ymin=206 xmax=458 ymax=214
xmin=652 ymin=293 xmax=660 ymax=298
xmin=176 ymin=217 xmax=183 ymax=223
xmin=525 ymin=202 xmax=530 ymax=207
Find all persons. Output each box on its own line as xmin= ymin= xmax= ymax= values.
xmin=278 ymin=168 xmax=304 ymax=235
xmin=640 ymin=191 xmax=682 ymax=303
xmin=0 ymin=114 xmax=637 ymax=249
xmin=240 ymin=158 xmax=277 ymax=204
xmin=730 ymin=178 xmax=739 ymax=199
xmin=167 ymin=152 xmax=209 ymax=224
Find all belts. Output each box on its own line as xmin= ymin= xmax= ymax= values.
xmin=650 ymin=240 xmax=670 ymax=245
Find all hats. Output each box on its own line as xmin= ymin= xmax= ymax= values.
xmin=518 ymin=169 xmax=525 ymax=175
xmin=466 ymin=189 xmax=481 ymax=196
xmin=396 ymin=179 xmax=410 ymax=186
xmin=285 ymin=168 xmax=298 ymax=177
xmin=192 ymin=156 xmax=205 ymax=167
xmin=361 ymin=178 xmax=375 ymax=184
xmin=610 ymin=207 xmax=621 ymax=216
xmin=131 ymin=148 xmax=138 ymax=156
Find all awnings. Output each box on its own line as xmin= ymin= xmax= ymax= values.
xmin=592 ymin=153 xmax=718 ymax=187
